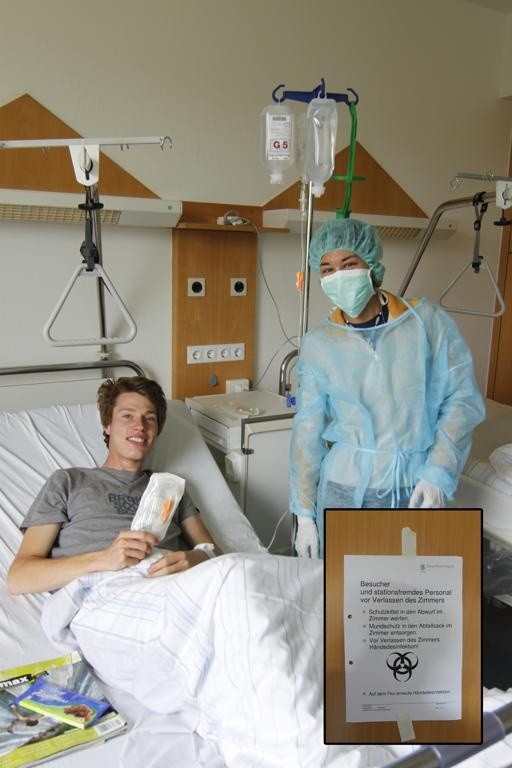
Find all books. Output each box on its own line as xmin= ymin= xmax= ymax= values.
xmin=0 ymin=651 xmax=128 ymax=768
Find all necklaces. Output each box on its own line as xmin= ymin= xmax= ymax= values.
xmin=340 ymin=307 xmax=383 ymax=345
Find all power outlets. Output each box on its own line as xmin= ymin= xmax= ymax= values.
xmin=186 ymin=342 xmax=245 ymax=364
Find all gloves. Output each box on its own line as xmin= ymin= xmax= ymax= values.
xmin=295 ymin=515 xmax=319 ymax=560
xmin=409 ymin=478 xmax=447 ymax=509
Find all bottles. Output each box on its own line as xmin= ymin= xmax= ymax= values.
xmin=304 ymin=98 xmax=337 ymax=202
xmin=260 ymin=103 xmax=296 ymax=184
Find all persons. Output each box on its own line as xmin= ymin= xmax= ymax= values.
xmin=7 ymin=703 xmax=47 ymax=735
xmin=285 ymin=212 xmax=486 ymax=562
xmin=4 ymin=372 xmax=227 ymax=599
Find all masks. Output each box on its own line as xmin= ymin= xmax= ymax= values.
xmin=319 ymin=265 xmax=376 ymax=318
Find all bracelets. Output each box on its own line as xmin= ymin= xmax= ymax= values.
xmin=192 ymin=541 xmax=218 ymax=559
